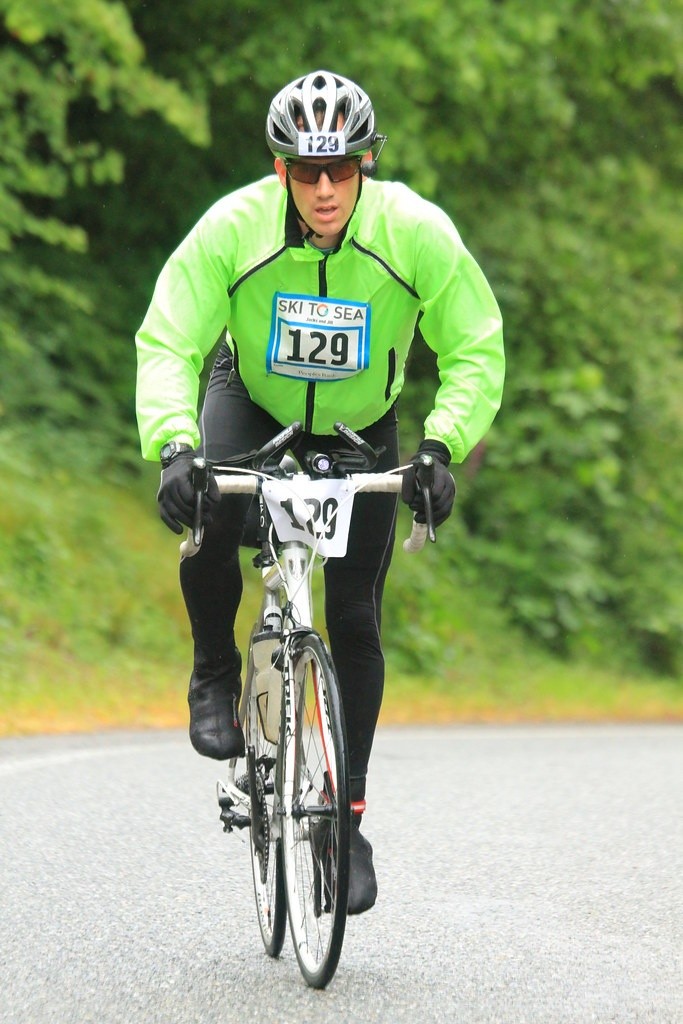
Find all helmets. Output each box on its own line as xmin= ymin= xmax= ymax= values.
xmin=264 ymin=70 xmax=376 ymax=158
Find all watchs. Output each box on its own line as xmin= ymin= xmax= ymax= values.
xmin=159 ymin=442 xmax=193 ymax=465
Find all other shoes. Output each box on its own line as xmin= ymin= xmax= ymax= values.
xmin=187 ymin=639 xmax=245 ymax=761
xmin=310 ymin=770 xmax=377 ymax=915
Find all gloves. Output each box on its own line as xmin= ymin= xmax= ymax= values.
xmin=402 ymin=440 xmax=456 ymax=527
xmin=157 ymin=451 xmax=221 ymax=534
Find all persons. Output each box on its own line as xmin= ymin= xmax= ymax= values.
xmin=134 ymin=71 xmax=506 ymax=916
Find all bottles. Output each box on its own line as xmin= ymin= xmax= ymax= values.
xmin=251 ymin=606 xmax=283 ymax=740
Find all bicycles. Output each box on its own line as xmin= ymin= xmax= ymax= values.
xmin=192 ymin=423 xmax=438 ymax=988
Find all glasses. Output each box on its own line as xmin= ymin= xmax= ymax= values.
xmin=283 ymin=155 xmax=362 ymax=184
